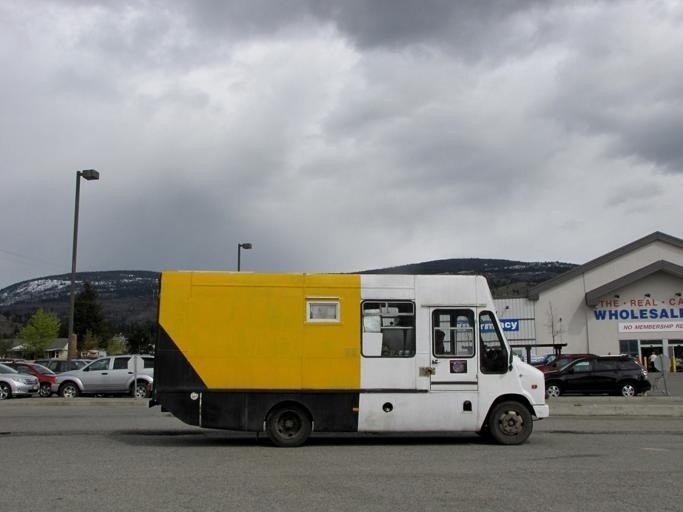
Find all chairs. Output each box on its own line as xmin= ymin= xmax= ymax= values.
xmin=436 ymin=331 xmax=453 ymax=355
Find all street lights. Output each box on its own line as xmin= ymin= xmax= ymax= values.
xmin=236 ymin=241 xmax=253 ymax=271
xmin=66 ymin=168 xmax=100 ymax=337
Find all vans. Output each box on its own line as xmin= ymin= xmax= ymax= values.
xmin=50 ymin=352 xmax=154 ymax=398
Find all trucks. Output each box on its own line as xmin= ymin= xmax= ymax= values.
xmin=148 ymin=271 xmax=550 ymax=447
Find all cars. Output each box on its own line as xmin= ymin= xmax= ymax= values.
xmin=531 ymin=354 xmax=554 ymax=365
xmin=0 ymin=363 xmax=41 ymax=400
xmin=543 ymin=355 xmax=653 ymax=399
xmin=0 ymin=357 xmax=102 ymax=377
xmin=0 ymin=361 xmax=55 ymax=398
xmin=534 ymin=354 xmax=648 ymax=377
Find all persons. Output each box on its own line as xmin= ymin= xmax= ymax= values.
xmin=648 ymin=352 xmax=657 ymax=372
xmin=436 ymin=331 xmax=445 ymax=354
